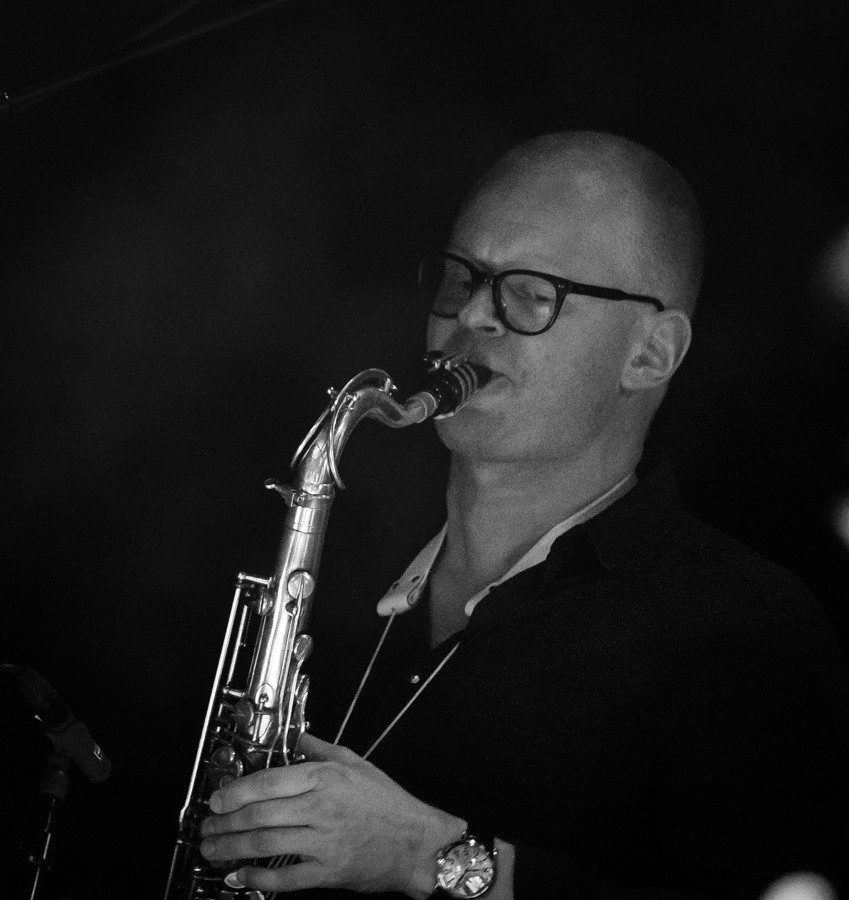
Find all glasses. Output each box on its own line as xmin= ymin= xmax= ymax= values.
xmin=416 ymin=252 xmax=664 ymax=336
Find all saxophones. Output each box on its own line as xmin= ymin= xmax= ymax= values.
xmin=162 ymin=346 xmax=492 ymax=900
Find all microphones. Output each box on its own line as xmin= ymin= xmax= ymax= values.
xmin=9 ymin=668 xmax=110 ymax=783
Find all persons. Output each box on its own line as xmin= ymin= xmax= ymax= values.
xmin=202 ymin=128 xmax=849 ymax=900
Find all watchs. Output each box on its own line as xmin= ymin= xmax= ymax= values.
xmin=426 ymin=819 xmax=506 ymax=900
